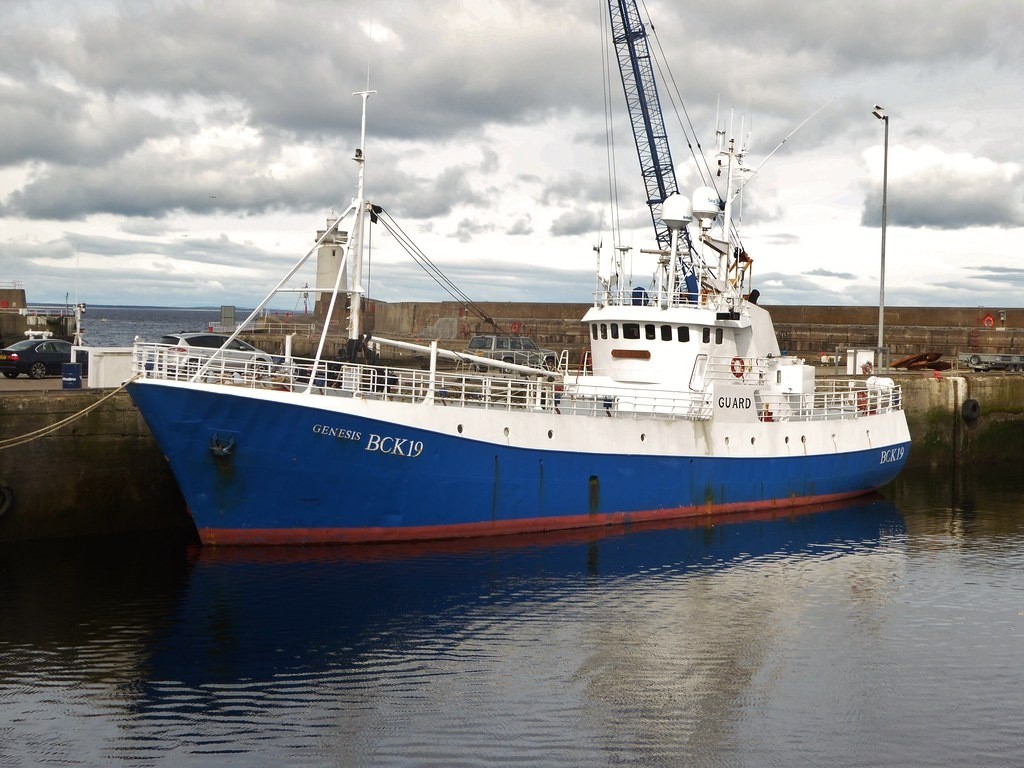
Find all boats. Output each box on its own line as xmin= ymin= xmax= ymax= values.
xmin=118 ymin=0 xmax=917 ymax=553
xmin=889 ymin=351 xmax=954 ymax=370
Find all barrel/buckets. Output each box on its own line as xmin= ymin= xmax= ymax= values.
xmin=62 ymin=363 xmax=82 ymax=389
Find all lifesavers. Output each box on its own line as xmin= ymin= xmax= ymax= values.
xmin=983 ymin=317 xmax=993 ymax=326
xmin=731 ymin=355 xmax=745 ymax=378
xmin=0 ymin=480 xmax=15 ymax=518
xmin=962 ymin=399 xmax=981 ymax=423
xmin=857 ymin=390 xmax=868 ymax=410
xmin=862 ymin=406 xmax=876 ymax=416
xmin=862 ymin=361 xmax=872 ymax=374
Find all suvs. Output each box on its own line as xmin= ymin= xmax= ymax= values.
xmin=137 ymin=332 xmax=272 ymax=384
xmin=463 ymin=335 xmax=559 ymax=377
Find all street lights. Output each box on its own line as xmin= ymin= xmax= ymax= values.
xmin=872 ymin=103 xmax=890 ymax=366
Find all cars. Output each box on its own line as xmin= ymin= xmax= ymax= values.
xmin=0 ymin=339 xmax=89 ymax=380
